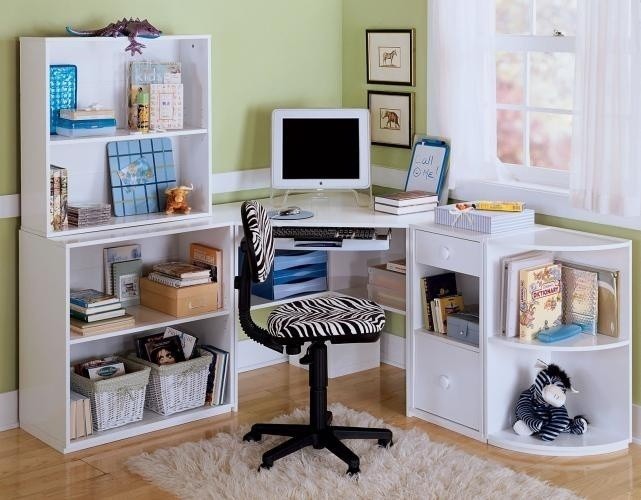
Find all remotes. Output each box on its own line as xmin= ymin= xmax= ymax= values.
xmin=278 ymin=207 xmax=300 ymax=216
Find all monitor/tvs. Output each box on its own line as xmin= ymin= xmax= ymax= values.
xmin=136 ymin=326 xmax=199 ymax=366
xmin=189 ymin=244 xmax=223 ymax=309
xmin=76 ymin=359 xmax=125 ymax=382
xmin=422 ymin=272 xmax=464 ymax=334
xmin=154 ymin=261 xmax=211 ymax=279
xmin=404 ymin=134 xmax=453 ymax=206
xmin=50 ymin=165 xmax=69 ymax=231
xmin=501 ymin=251 xmax=621 ymax=341
xmin=374 ymin=191 xmax=439 ymax=215
xmin=68 ymin=202 xmax=111 ymax=228
xmin=200 ymin=345 xmax=229 ymax=406
xmin=70 ymin=288 xmax=136 ymax=336
xmin=148 ymin=272 xmax=213 ymax=287
xmin=104 ymin=245 xmax=142 ymax=308
xmin=70 ymin=390 xmax=93 ymax=441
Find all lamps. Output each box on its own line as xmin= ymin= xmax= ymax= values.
xmin=233 ymin=200 xmax=393 ymax=482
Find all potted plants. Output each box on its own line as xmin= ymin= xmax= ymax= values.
xmin=367 ymin=90 xmax=415 ymax=148
xmin=366 ymin=28 xmax=416 ymax=86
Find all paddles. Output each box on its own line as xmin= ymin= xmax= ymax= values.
xmin=124 ymin=402 xmax=583 ymax=500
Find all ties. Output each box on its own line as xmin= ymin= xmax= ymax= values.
xmin=272 ymin=109 xmax=371 ymax=190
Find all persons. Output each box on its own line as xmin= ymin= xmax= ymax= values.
xmin=150 ymin=341 xmax=179 ymax=366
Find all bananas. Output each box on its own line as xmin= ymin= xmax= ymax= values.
xmin=273 ymin=226 xmax=375 ymax=240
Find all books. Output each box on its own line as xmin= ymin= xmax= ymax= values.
xmin=368 ymin=266 xmax=406 ymax=289
xmin=447 ymin=307 xmax=478 ymax=344
xmin=380 ymin=332 xmax=405 ymax=369
xmin=140 ymin=278 xmax=218 ymax=318
xmin=288 ymin=335 xmax=381 ymax=378
xmin=367 ymin=285 xmax=405 ymax=310
xmin=380 ymin=306 xmax=405 ymax=338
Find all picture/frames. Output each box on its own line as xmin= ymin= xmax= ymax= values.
xmin=211 ymin=178 xmax=445 ymax=225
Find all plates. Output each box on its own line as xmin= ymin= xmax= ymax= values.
xmin=20 ymin=34 xmax=213 ymax=237
xmin=18 ymin=194 xmax=228 ymax=453
xmin=406 ymin=226 xmax=632 ymax=455
